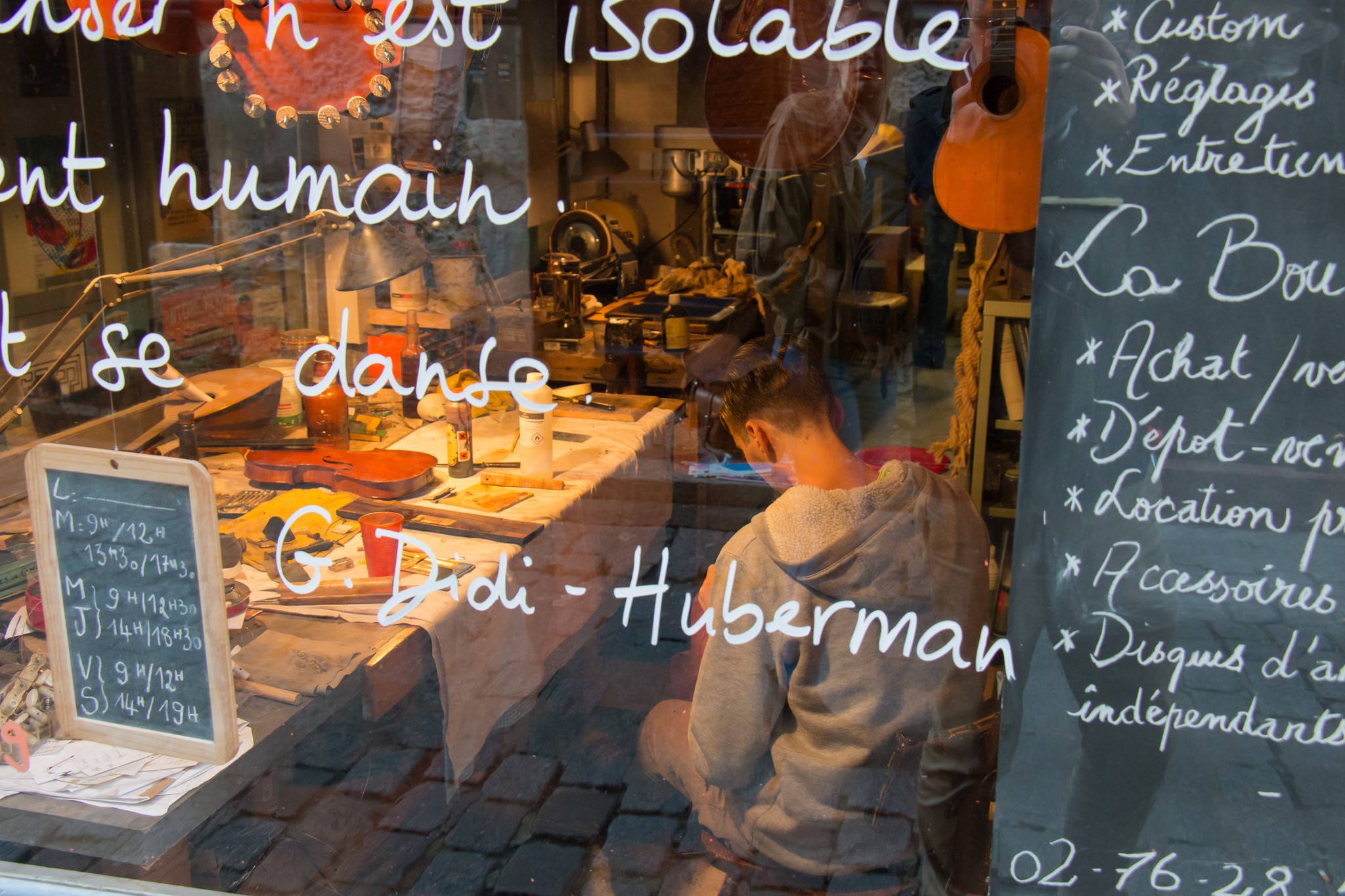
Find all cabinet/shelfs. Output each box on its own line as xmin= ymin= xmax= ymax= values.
xmin=971 ymin=301 xmax=1031 ymax=518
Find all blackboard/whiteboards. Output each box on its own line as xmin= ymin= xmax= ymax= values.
xmin=24 ymin=442 xmax=240 ymax=764
xmin=986 ymin=0 xmax=1345 ymax=896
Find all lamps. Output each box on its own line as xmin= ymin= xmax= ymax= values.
xmin=0 ymin=195 xmax=431 ymax=434
xmin=426 ymin=119 xmax=629 ymax=307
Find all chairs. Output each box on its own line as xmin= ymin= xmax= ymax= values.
xmin=701 ymin=712 xmax=1000 ymax=896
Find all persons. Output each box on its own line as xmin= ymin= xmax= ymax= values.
xmin=731 ymin=0 xmax=925 ymax=469
xmin=635 ymin=338 xmax=992 ymax=896
xmin=996 ymin=0 xmax=1178 ymax=896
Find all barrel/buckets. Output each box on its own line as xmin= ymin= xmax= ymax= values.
xmin=258 ymin=359 xmax=303 ymax=427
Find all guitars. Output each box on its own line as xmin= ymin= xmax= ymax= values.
xmin=933 ymin=0 xmax=1049 ymax=233
xmin=704 ymin=0 xmax=887 ymax=173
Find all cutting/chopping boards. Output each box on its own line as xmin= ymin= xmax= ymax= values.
xmin=552 ymin=392 xmax=661 ymax=422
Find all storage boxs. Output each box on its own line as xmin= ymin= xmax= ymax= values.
xmin=865 ymin=226 xmax=911 ymax=340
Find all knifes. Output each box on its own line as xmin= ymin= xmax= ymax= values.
xmin=552 ymin=394 xmax=616 ymax=411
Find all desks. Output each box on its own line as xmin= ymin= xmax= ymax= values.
xmin=466 ymin=314 xmax=741 ymax=402
xmin=0 ymin=391 xmax=688 ymax=886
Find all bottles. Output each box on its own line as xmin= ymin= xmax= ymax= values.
xmin=661 ymin=294 xmax=690 ymax=353
xmin=517 ymin=372 xmax=553 ymax=476
xmin=445 ymin=387 xmax=474 ymax=478
xmin=178 ymin=410 xmax=209 ymax=470
xmin=280 ymin=329 xmax=322 ymax=409
xmin=304 ymin=335 xmax=350 ymax=452
xmin=400 ymin=309 xmax=427 ymax=419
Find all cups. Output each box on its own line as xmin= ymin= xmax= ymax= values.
xmin=359 ymin=511 xmax=404 ymax=577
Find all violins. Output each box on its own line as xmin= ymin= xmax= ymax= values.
xmin=197 ymin=438 xmax=439 ymax=500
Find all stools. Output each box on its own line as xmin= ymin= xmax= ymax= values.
xmin=837 ymin=290 xmax=908 ymax=399
